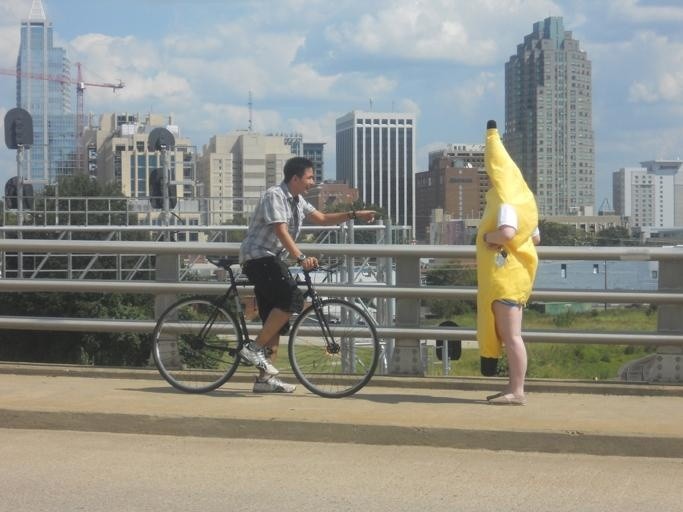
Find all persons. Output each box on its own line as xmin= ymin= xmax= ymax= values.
xmin=475 ymin=119 xmax=544 ymax=409
xmin=236 ymin=157 xmax=377 ymax=395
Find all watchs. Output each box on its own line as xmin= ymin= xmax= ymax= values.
xmin=296 ymin=254 xmax=305 ymax=264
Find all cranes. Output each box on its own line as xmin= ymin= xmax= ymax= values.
xmin=0 ymin=60 xmax=129 ymax=197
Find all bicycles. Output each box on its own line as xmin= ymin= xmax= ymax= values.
xmin=150 ymin=255 xmax=382 ymax=398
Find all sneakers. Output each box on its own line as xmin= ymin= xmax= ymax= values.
xmin=251 ymin=377 xmax=296 ymax=394
xmin=237 ymin=342 xmax=280 ymax=376
xmin=486 ymin=391 xmax=527 ymax=406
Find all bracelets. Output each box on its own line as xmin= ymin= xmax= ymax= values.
xmin=348 ymin=210 xmax=356 ymax=220
